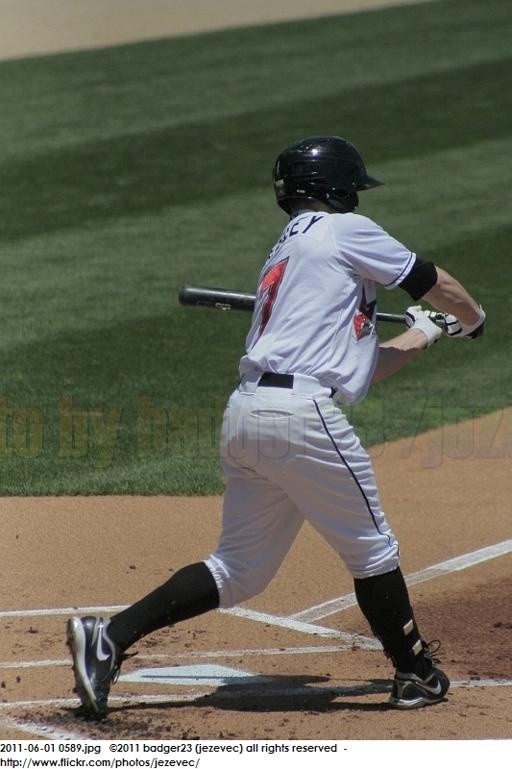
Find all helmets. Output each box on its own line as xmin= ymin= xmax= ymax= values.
xmin=270 ymin=136 xmax=386 ymax=211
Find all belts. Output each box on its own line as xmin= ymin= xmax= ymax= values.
xmin=239 ymin=371 xmax=336 ymax=397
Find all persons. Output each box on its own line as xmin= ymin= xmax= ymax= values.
xmin=64 ymin=135 xmax=488 ymax=722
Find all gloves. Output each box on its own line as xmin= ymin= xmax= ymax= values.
xmin=442 ymin=304 xmax=486 ymax=341
xmin=406 ymin=306 xmax=450 ymax=349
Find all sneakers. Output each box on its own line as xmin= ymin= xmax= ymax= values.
xmin=389 ymin=663 xmax=452 ymax=709
xmin=66 ymin=615 xmax=125 ymax=719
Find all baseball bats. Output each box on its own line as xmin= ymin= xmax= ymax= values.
xmin=179 ymin=283 xmax=448 ymax=323
